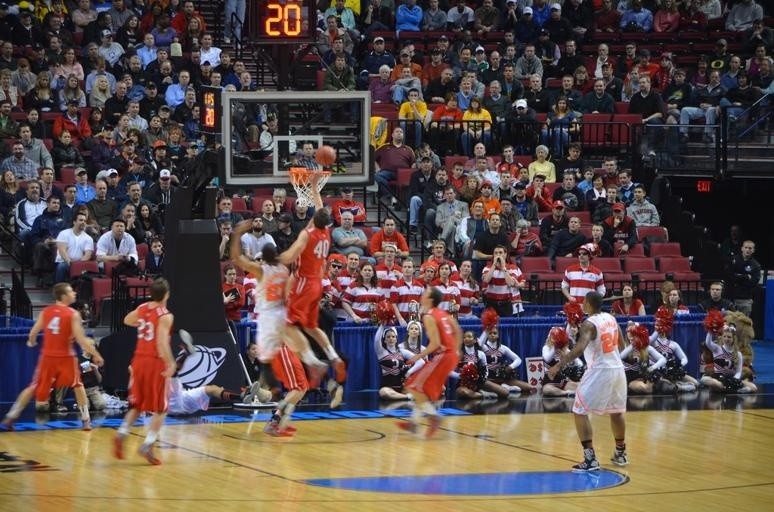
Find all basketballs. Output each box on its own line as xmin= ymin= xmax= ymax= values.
xmin=316 ymin=145 xmax=336 ymax=165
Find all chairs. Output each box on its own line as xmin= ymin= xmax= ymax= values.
xmin=0 ymin=29 xmax=773 ymax=319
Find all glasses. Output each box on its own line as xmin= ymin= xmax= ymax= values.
xmin=332 ymin=264 xmax=342 ymax=269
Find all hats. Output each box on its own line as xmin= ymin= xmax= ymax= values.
xmin=612 ymin=204 xmax=625 ymax=212
xmin=553 ymin=200 xmax=565 ymax=209
xmin=523 ymin=3 xmax=561 ymax=14
xmin=478 ymin=181 xmax=525 ymax=204
xmin=190 ymin=141 xmax=198 ymax=148
xmin=74 ymin=167 xmax=118 ymax=180
xmin=155 ymin=141 xmax=166 ymax=149
xmin=516 ymin=100 xmax=527 ymax=109
xmin=160 ymin=169 xmax=169 ymax=178
xmin=576 ymin=245 xmax=593 ymax=260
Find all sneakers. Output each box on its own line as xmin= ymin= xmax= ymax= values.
xmin=138 ymin=445 xmax=160 ymax=465
xmin=179 ymin=328 xmax=195 ymax=354
xmin=572 ymin=457 xmax=600 ymax=472
xmin=116 ymin=434 xmax=125 ymax=459
xmin=266 ymin=417 xmax=297 ymax=436
xmin=395 ymin=415 xmax=442 ymax=438
xmin=308 ymin=358 xmax=348 ymax=389
xmin=610 ymin=455 xmax=627 ymax=465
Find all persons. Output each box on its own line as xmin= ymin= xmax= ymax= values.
xmin=3 ymin=282 xmax=106 ymax=432
xmin=114 ymin=277 xmax=175 ymax=465
xmin=392 ymin=287 xmax=464 ymax=440
xmin=0 ymin=0 xmax=773 ymax=416
xmin=548 ymin=289 xmax=630 ymax=471
xmin=265 ymin=342 xmax=311 ymax=439
